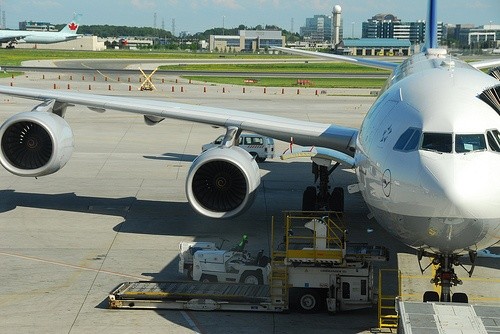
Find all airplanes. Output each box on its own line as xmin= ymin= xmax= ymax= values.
xmin=0 ymin=19 xmax=81 ymax=49
xmin=0 ymin=0 xmax=500 ymax=305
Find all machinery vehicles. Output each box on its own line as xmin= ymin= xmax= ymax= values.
xmin=106 ymin=212 xmax=390 ymax=314
